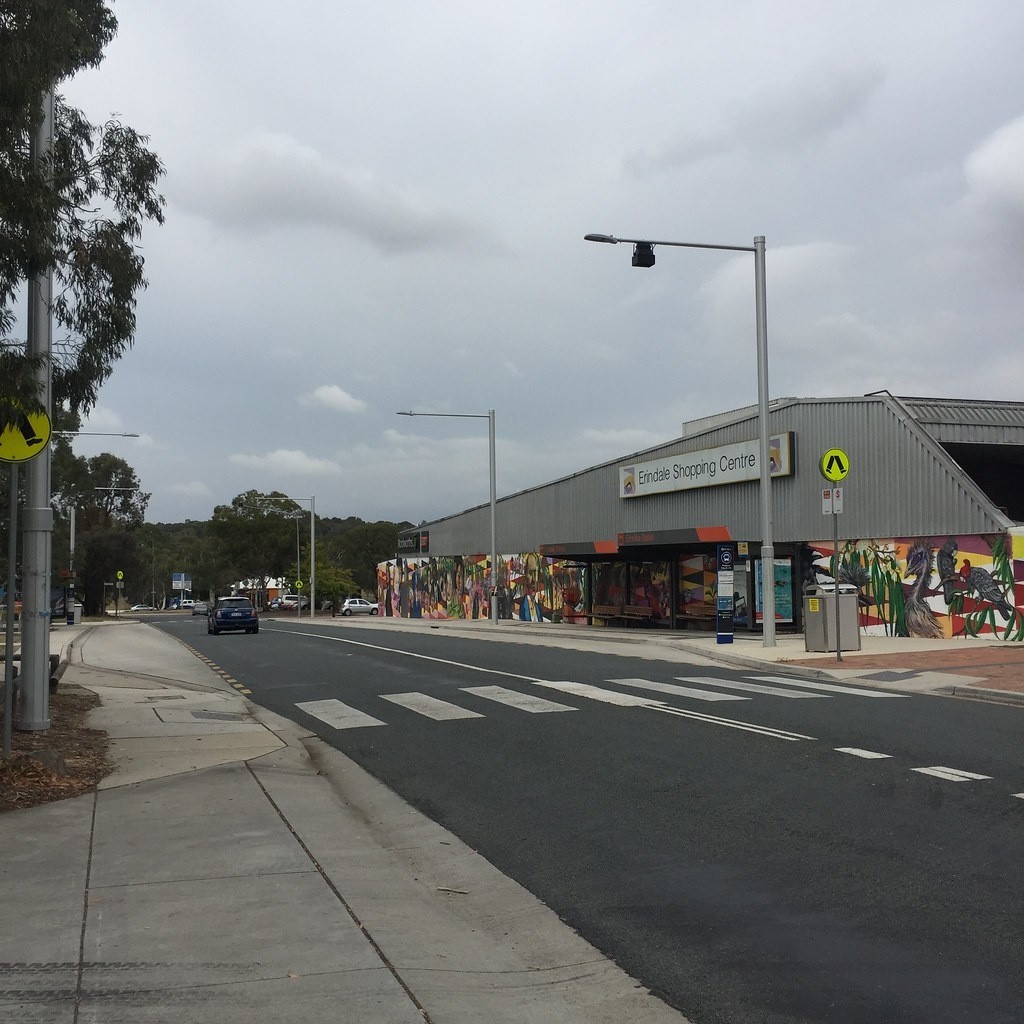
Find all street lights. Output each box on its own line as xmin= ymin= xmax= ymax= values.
xmin=67 ymin=483 xmax=140 ymax=626
xmin=584 ymin=231 xmax=779 ymax=651
xmin=254 ymin=494 xmax=317 ymax=619
xmin=103 ymin=582 xmax=113 ymax=617
xmin=4 ymin=428 xmax=141 ymax=753
xmin=395 ymin=408 xmax=498 ymax=625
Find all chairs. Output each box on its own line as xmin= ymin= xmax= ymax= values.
xmin=615 ymin=605 xmax=654 ymax=627
xmin=675 ymin=603 xmax=717 ymax=631
xmin=586 ymin=604 xmax=622 ymax=626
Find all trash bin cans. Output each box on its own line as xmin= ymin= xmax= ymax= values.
xmin=802 ymin=584 xmax=862 ymax=653
xmin=73 ymin=603 xmax=83 ymax=625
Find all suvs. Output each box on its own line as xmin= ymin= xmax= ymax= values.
xmin=339 ymin=599 xmax=378 ymax=616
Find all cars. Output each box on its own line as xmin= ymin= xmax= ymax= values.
xmin=170 ymin=598 xmax=214 ymax=616
xmin=207 ymin=596 xmax=258 ymax=635
xmin=267 ymin=594 xmax=341 ymax=612
xmin=130 ymin=603 xmax=158 ymax=612
